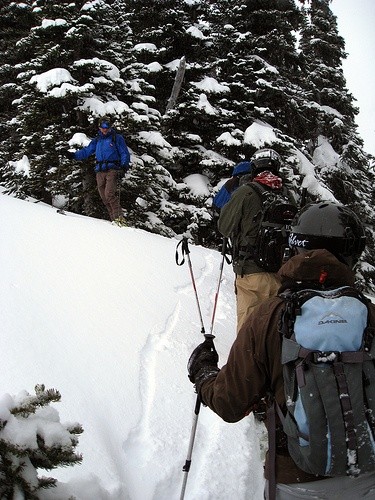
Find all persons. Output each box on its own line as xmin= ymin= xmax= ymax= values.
xmin=214 ymin=162 xmax=254 ymax=209
xmin=187 ymin=200 xmax=375 ymax=500
xmin=67 ymin=121 xmax=130 ymax=221
xmin=217 ymin=149 xmax=299 ymax=334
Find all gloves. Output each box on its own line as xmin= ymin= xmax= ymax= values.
xmin=118 ymin=167 xmax=126 ymax=178
xmin=187 ymin=338 xmax=222 ymax=392
xmin=60 ymin=148 xmax=74 ymax=160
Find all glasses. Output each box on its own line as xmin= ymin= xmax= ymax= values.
xmin=99 ymin=123 xmax=110 ymax=129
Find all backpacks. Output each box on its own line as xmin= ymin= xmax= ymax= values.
xmin=242 ymin=182 xmax=299 ymax=272
xmin=269 ymin=283 xmax=375 ymax=478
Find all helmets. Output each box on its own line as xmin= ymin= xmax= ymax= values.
xmin=232 ymin=162 xmax=252 ymax=175
xmin=286 ymin=199 xmax=366 ymax=252
xmin=250 ymin=149 xmax=281 ymax=168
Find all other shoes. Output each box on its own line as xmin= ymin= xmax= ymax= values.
xmin=113 ymin=216 xmax=126 ymax=226
